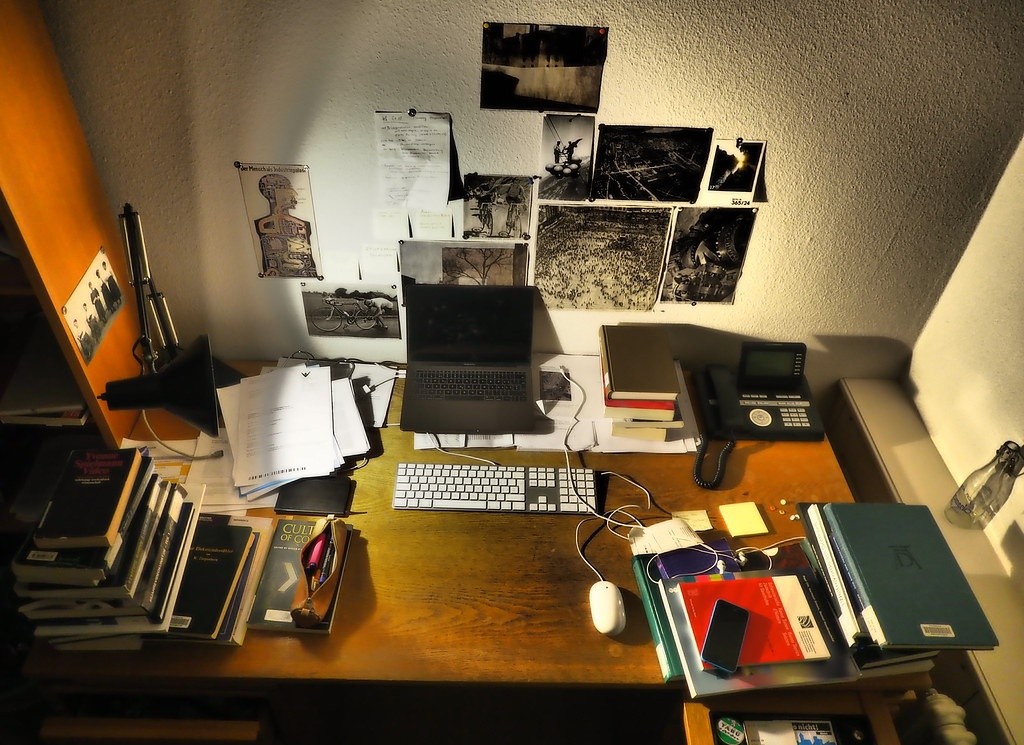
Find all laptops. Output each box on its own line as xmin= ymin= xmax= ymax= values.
xmin=399 ymin=285 xmax=535 ymax=434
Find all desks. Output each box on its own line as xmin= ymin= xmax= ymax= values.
xmin=24 ymin=374 xmax=931 ymax=691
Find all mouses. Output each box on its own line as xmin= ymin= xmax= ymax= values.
xmin=590 ymin=580 xmax=626 ymax=634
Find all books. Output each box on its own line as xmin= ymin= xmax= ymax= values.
xmin=631 ymin=500 xmax=999 ymax=700
xmin=10 ymin=443 xmax=355 ymax=658
xmin=598 ymin=324 xmax=684 ymax=444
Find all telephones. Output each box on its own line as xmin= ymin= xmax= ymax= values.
xmin=674 ymin=342 xmax=826 ymax=442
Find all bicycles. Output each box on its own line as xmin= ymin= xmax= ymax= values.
xmin=311 ymin=291 xmax=376 ymax=332
xmin=480 ymin=202 xmax=498 ymax=235
xmin=505 ymin=203 xmax=527 ymax=237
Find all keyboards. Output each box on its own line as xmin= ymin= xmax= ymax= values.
xmin=392 ymin=463 xmax=599 ymax=515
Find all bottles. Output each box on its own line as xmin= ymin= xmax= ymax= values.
xmin=945 ymin=440 xmax=1021 ymax=527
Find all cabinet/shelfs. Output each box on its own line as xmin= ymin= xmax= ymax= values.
xmin=1 ymin=0 xmax=154 ymax=455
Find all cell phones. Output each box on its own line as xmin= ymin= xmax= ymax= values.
xmin=701 ymin=599 xmax=750 ymax=673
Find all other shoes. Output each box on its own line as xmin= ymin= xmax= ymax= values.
xmin=372 ymin=324 xmax=381 ymax=328
xmin=380 ymin=326 xmax=388 ymax=328
xmin=506 ymin=220 xmax=511 ymax=226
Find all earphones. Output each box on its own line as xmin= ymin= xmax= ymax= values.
xmin=717 ymin=559 xmax=726 ymax=574
xmin=734 ymin=555 xmax=748 ymax=567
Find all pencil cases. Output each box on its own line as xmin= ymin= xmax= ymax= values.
xmin=291 ymin=514 xmax=347 ymax=629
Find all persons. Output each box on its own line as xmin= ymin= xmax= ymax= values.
xmin=553 ymin=140 xmax=574 ymax=164
xmin=464 ymin=177 xmax=526 ymax=231
xmin=73 ymin=261 xmax=122 ymax=361
xmin=363 ymin=298 xmax=393 ymax=328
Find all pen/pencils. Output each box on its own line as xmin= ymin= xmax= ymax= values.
xmin=305 ymin=527 xmax=335 ymax=591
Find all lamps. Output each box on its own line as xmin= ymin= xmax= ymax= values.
xmin=95 ymin=200 xmax=246 ymax=437
